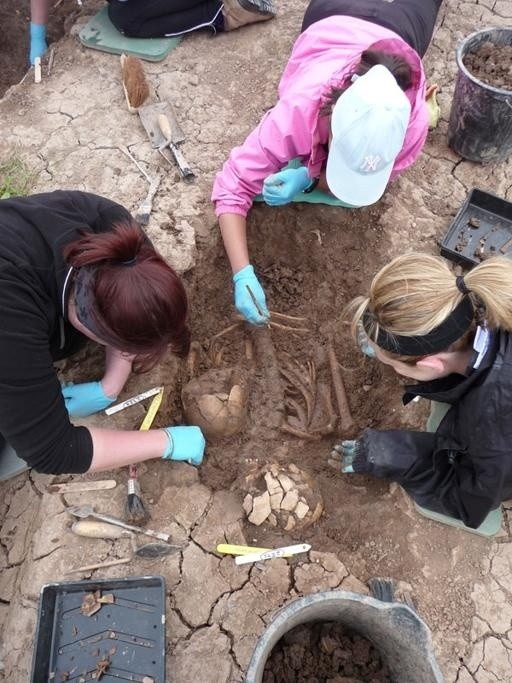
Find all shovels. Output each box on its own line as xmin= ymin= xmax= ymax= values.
xmin=68 ymin=504 xmax=171 ymax=542
xmin=139 ymin=101 xmax=195 ymax=182
xmin=71 ymin=521 xmax=182 ymax=558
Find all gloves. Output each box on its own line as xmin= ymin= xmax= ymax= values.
xmin=62 ymin=381 xmax=117 ymax=418
xmin=263 ymin=166 xmax=315 ymax=207
xmin=232 ymin=264 xmax=271 ymax=327
xmin=30 ymin=24 xmax=47 ymax=63
xmin=328 ymin=440 xmax=354 ymax=474
xmin=162 ymin=425 xmax=205 ymax=465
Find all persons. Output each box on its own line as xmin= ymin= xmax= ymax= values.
xmin=0 ymin=190 xmax=206 ymax=480
xmin=207 ymin=1 xmax=447 ymax=329
xmin=321 ymin=249 xmax=511 ymax=530
xmin=27 ymin=1 xmax=278 ymax=67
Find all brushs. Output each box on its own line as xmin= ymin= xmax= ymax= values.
xmin=136 ymin=175 xmax=160 ymax=225
xmin=126 ymin=464 xmax=146 ymax=522
xmin=370 ymin=578 xmax=392 ymax=604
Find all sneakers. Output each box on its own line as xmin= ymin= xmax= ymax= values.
xmin=221 ymin=0 xmax=276 ymax=32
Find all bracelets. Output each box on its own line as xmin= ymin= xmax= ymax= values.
xmin=303 ymin=175 xmax=321 ymax=195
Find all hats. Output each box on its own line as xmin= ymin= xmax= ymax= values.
xmin=325 ymin=64 xmax=412 ymax=207
